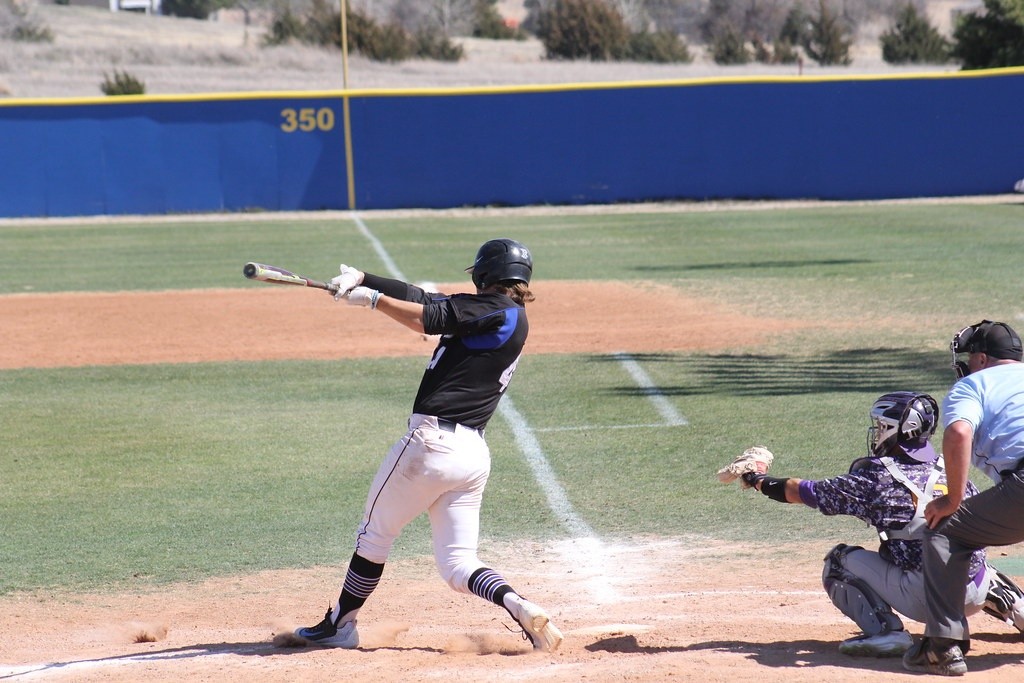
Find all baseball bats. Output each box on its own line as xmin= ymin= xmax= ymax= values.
xmin=242 ymin=261 xmax=339 ymax=293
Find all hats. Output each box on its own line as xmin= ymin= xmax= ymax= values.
xmin=950 ymin=319 xmax=1023 ymax=362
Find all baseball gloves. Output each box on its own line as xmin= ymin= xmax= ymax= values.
xmin=716 ymin=445 xmax=774 ymax=490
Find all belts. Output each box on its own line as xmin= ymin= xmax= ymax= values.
xmin=408 ymin=416 xmax=484 ymax=437
xmin=974 ymin=565 xmax=986 ymax=588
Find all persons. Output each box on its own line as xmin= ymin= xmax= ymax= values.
xmin=903 ymin=319 xmax=1024 ymax=674
xmin=296 ymin=240 xmax=563 ymax=651
xmin=718 ymin=392 xmax=1024 ymax=654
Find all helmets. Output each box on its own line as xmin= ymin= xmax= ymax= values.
xmin=867 ymin=391 xmax=939 ymax=457
xmin=464 ymin=239 xmax=533 ymax=291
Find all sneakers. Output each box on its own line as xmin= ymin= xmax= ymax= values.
xmin=502 ymin=601 xmax=563 ymax=652
xmin=838 ymin=629 xmax=913 ymax=658
xmin=295 ymin=600 xmax=360 ymax=649
xmin=903 ymin=637 xmax=967 ymax=676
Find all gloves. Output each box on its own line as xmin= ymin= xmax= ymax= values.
xmin=741 ymin=470 xmax=767 ymax=491
xmin=329 ymin=263 xmax=365 ymax=301
xmin=343 ymin=285 xmax=384 ymax=310
